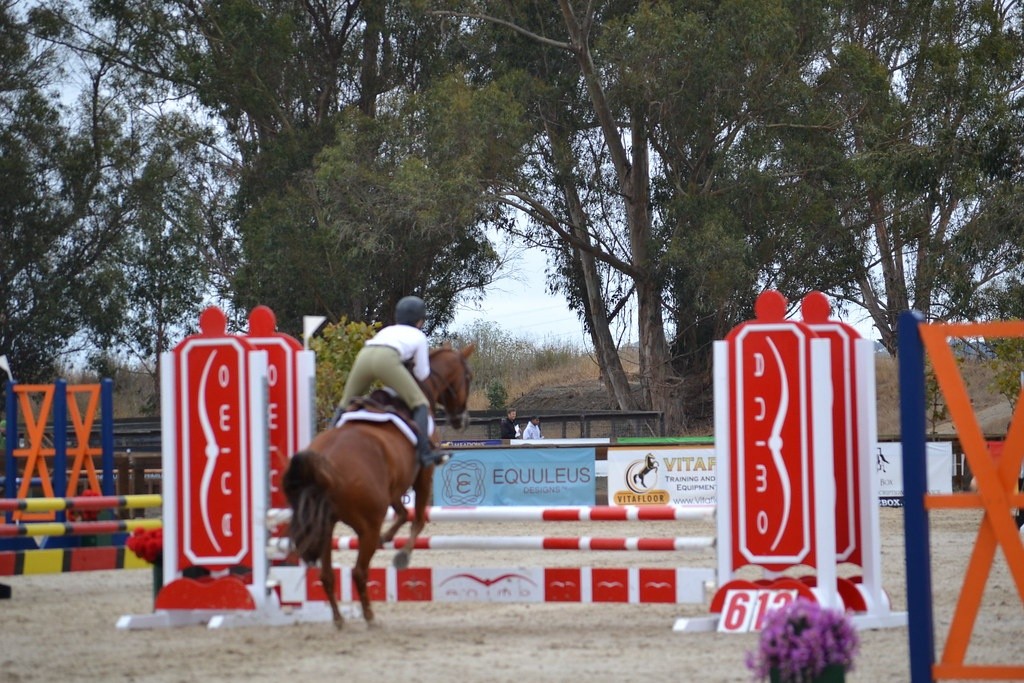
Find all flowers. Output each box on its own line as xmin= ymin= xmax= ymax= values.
xmin=745 ymin=596 xmax=860 ymax=683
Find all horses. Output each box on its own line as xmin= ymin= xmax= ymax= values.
xmin=280 ymin=339 xmax=477 ymax=629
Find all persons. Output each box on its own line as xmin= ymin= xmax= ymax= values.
xmin=1006 ymin=421 xmax=1024 ymax=530
xmin=523 ymin=415 xmax=541 ymax=439
xmin=500 ymin=408 xmax=521 ymax=439
xmin=330 ymin=296 xmax=454 ymax=467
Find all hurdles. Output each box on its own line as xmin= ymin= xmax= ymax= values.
xmin=0 ymin=290 xmax=908 ymax=635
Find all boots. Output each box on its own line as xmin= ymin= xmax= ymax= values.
xmin=330 ymin=408 xmax=344 ymax=428
xmin=413 ymin=403 xmax=454 ymax=466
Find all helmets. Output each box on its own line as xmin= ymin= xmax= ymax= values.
xmin=395 ymin=296 xmax=427 ymax=322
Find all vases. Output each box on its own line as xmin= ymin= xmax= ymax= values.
xmin=770 ymin=664 xmax=844 ymax=683
